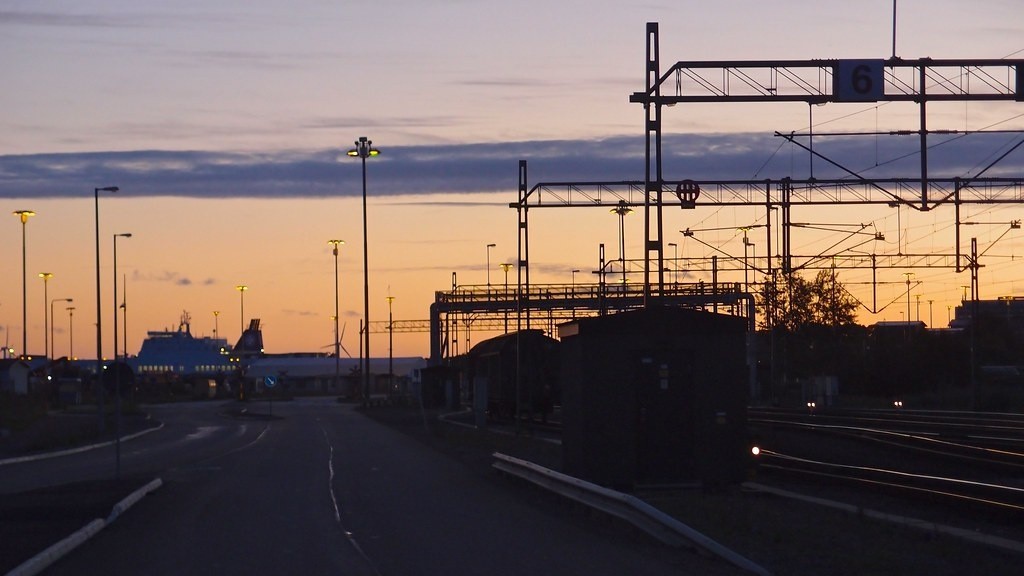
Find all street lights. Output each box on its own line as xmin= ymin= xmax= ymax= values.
xmin=487 ymin=243 xmax=497 ymax=284
xmin=770 ymin=207 xmax=779 ymax=255
xmin=236 ymin=285 xmax=248 ymax=333
xmin=327 ymin=239 xmax=346 ymax=357
xmin=51 ymin=298 xmax=73 ymax=360
xmin=346 ymin=137 xmax=381 ymax=395
xmin=39 ymin=272 xmax=53 ymax=358
xmin=498 ymin=264 xmax=515 ymax=335
xmin=900 ymin=310 xmax=904 ymax=321
xmin=112 ymin=233 xmax=132 ymax=360
xmin=748 ymin=243 xmax=756 ymax=282
xmin=212 ymin=310 xmax=221 ymax=339
xmin=668 ymin=242 xmax=677 ymax=284
xmin=904 ymin=271 xmax=970 ymax=328
xmin=94 ymin=186 xmax=120 ymax=360
xmin=12 ymin=211 xmax=36 ymax=354
xmin=611 ymin=201 xmax=634 ymax=312
xmin=386 ymin=292 xmax=398 ymax=394
xmin=736 ymin=226 xmax=752 ymax=317
xmin=65 ymin=308 xmax=75 ymax=360
